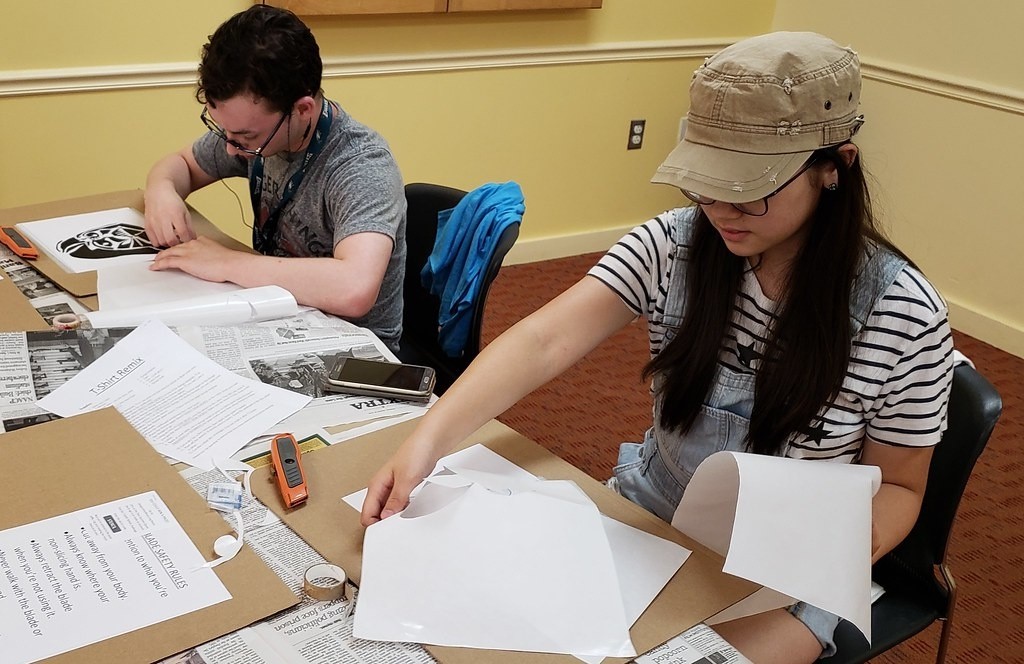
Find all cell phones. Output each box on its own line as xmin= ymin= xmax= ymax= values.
xmin=326 ymin=356 xmax=437 ymax=402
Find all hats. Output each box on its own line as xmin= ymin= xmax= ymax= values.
xmin=650 ymin=30 xmax=862 ymax=204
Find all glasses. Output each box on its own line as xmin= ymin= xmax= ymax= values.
xmin=200 ymin=92 xmax=311 ymax=158
xmin=681 ymin=155 xmax=824 ymax=217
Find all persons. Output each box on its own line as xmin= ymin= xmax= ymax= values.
xmin=362 ymin=30 xmax=953 ymax=664
xmin=145 ymin=4 xmax=408 ymax=363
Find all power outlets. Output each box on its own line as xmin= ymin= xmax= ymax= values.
xmin=628 ymin=119 xmax=646 ymax=150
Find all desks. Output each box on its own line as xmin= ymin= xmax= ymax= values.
xmin=0 ymin=192 xmax=760 ymax=664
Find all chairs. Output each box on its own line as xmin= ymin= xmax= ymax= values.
xmin=401 ymin=182 xmax=521 ymax=397
xmin=800 ymin=362 xmax=1003 ymax=664
